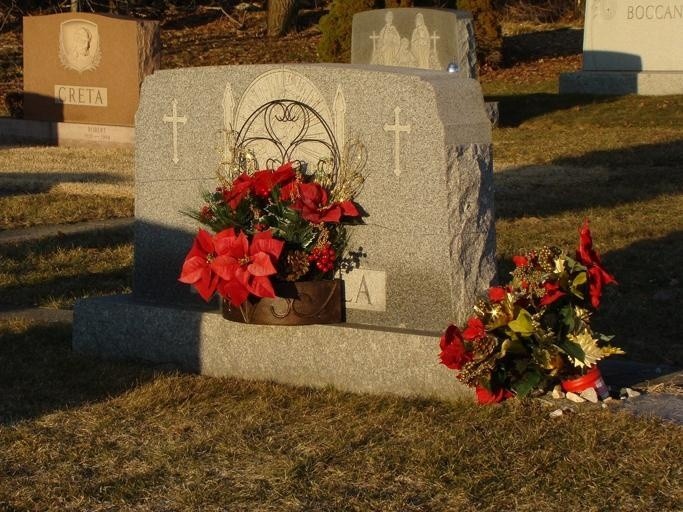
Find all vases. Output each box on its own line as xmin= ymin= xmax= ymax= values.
xmin=221 ymin=278 xmax=343 ymax=325
xmin=560 ymin=367 xmax=609 ymax=399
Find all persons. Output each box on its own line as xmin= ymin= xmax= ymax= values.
xmin=69 ymin=27 xmax=94 ymax=66
xmin=376 ymin=11 xmax=431 ymax=68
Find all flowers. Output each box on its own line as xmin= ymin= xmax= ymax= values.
xmin=437 ymin=219 xmax=624 ymax=405
xmin=178 ymin=162 xmax=366 ymax=306
